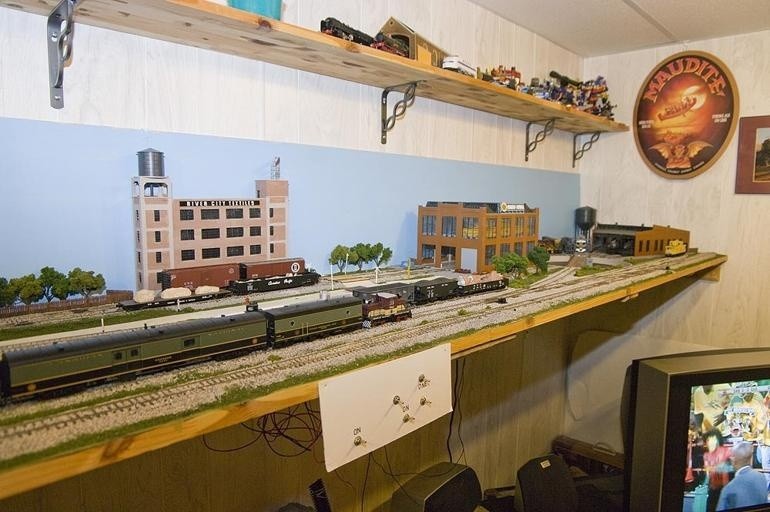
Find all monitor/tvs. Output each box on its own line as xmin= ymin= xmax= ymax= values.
xmin=620 ymin=347 xmax=770 ymax=512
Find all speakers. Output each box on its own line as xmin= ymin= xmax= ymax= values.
xmin=392 ymin=462 xmax=483 ymax=512
xmin=513 ymin=454 xmax=580 ymax=512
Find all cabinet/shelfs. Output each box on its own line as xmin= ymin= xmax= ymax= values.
xmin=1 ymin=0 xmax=728 ymax=501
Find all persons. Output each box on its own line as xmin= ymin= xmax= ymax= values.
xmin=685 ymin=380 xmax=770 ymax=512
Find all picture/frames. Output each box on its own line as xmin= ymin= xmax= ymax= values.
xmin=734 ymin=115 xmax=770 ymax=194
